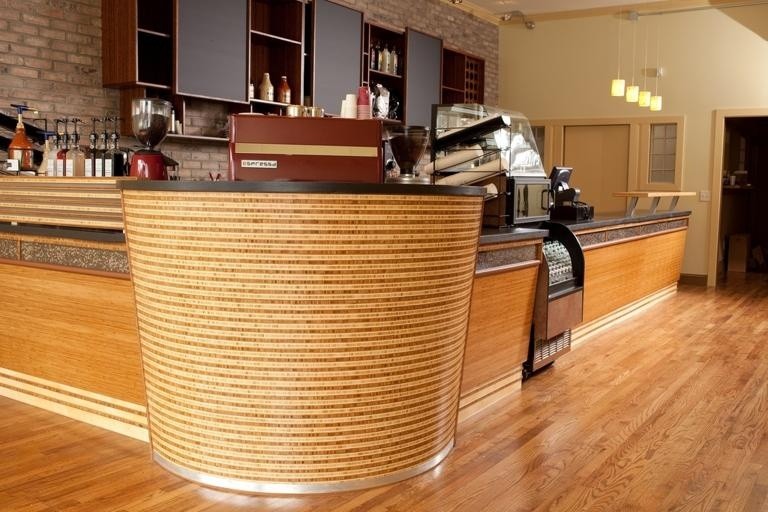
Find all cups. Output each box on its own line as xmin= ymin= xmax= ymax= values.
xmin=424 ymin=113 xmax=513 ymax=199
xmin=339 ymin=86 xmax=372 ymax=119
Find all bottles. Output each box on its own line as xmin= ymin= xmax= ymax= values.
xmin=277 ymin=75 xmax=292 ymax=104
xmin=258 ymin=71 xmax=275 ymax=102
xmin=250 ymin=79 xmax=254 ymax=100
xmin=370 ymin=39 xmax=405 ymax=77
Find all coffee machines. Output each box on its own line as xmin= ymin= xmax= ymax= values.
xmin=384 ymin=124 xmax=432 ymax=184
xmin=129 ymin=97 xmax=179 ymax=181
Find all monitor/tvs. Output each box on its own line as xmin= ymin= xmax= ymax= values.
xmin=549 ymin=166 xmax=572 ymax=193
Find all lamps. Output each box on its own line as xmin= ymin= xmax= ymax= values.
xmin=609 ymin=2 xmax=663 ymax=114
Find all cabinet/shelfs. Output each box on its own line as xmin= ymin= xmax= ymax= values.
xmin=305 ymin=1 xmax=486 ymax=142
xmin=101 ymin=0 xmax=305 ymax=143
